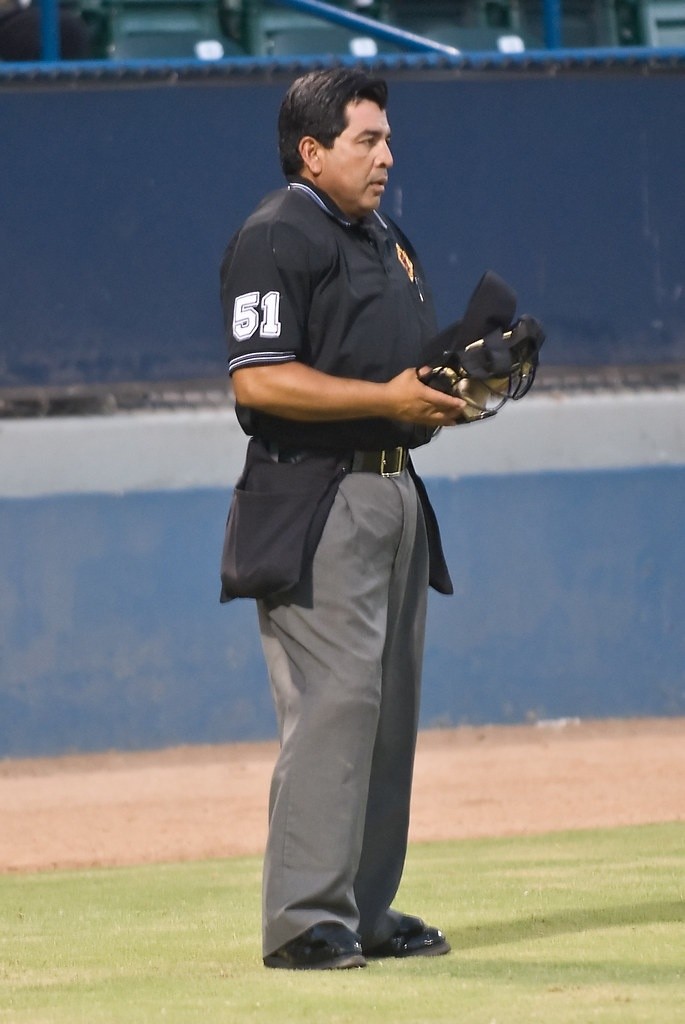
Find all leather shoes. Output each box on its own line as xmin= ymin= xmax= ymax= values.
xmin=263 ymin=921 xmax=367 ymax=969
xmin=362 ymin=914 xmax=450 ymax=957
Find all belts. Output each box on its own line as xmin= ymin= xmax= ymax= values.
xmin=351 ymin=447 xmax=409 ymax=476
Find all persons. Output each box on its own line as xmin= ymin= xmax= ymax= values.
xmin=220 ymin=70 xmax=467 ymax=966
